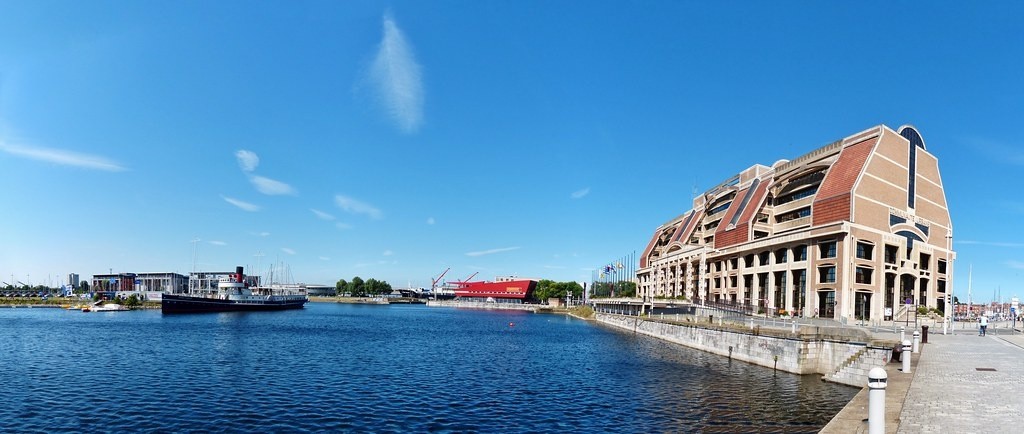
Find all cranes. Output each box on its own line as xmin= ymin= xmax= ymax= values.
xmin=431 ymin=267 xmax=451 ymax=290
xmin=457 ymin=271 xmax=478 ymax=288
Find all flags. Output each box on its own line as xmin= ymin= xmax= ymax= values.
xmin=599 ymin=261 xmax=625 ymax=279
xmin=133 ymin=280 xmax=140 ymax=284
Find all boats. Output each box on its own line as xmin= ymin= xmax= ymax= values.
xmin=161 ymin=292 xmax=309 ymax=316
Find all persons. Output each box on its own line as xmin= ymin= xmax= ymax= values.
xmin=779 ymin=306 xmax=796 ymax=318
xmin=979 ymin=314 xmax=989 ymax=336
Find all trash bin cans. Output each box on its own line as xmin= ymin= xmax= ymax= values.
xmin=709 ymin=315 xmax=713 ymax=323
